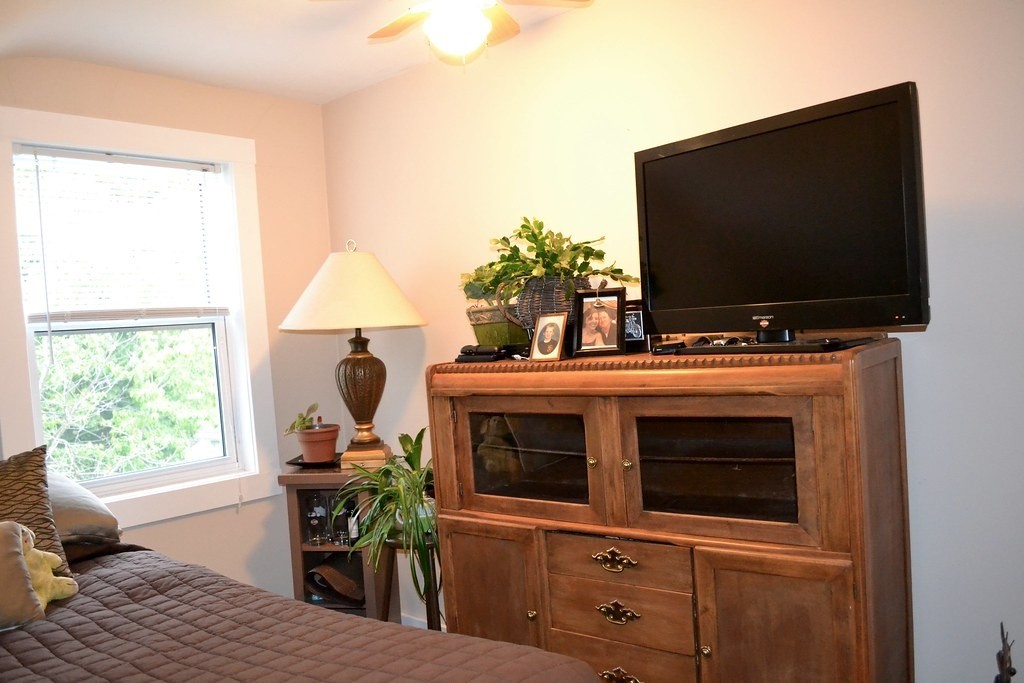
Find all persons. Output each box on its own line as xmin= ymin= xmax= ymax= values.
xmin=582 ymin=307 xmax=615 ymax=347
xmin=538 ymin=324 xmax=558 ymax=355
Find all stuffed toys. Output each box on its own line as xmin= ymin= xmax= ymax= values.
xmin=19 ymin=524 xmax=79 ymax=610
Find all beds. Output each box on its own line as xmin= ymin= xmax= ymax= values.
xmin=0 ymin=532 xmax=601 ymax=683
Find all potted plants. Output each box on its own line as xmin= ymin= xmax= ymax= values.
xmin=283 ymin=401 xmax=340 ymax=461
xmin=333 ymin=426 xmax=448 ymax=626
xmin=456 ymin=255 xmax=531 ymax=346
xmin=484 ymin=215 xmax=641 ymax=329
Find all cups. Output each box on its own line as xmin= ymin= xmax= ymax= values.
xmin=306 ymin=494 xmax=349 ymax=545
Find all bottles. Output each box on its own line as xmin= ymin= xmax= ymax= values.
xmin=345 ymin=500 xmax=360 ymax=547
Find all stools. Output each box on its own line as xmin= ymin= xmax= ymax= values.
xmin=385 ymin=530 xmax=440 ymax=631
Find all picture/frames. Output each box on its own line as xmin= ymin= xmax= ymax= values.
xmin=626 ymin=299 xmax=648 ymax=354
xmin=528 ymin=312 xmax=569 ymax=362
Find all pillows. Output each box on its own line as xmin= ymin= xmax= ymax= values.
xmin=47 ymin=474 xmax=118 ymax=534
xmin=0 ymin=444 xmax=73 ymax=580
xmin=0 ymin=521 xmax=46 ymax=626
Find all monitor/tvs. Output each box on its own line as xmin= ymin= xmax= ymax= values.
xmin=633 ymin=80 xmax=930 ymax=355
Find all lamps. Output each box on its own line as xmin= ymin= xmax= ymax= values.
xmin=278 ymin=238 xmax=429 ymax=470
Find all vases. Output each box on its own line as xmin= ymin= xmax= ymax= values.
xmin=572 ymin=287 xmax=626 ymax=357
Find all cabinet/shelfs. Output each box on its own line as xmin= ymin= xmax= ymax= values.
xmin=277 ymin=464 xmax=402 ymax=624
xmin=424 ymin=332 xmax=916 ymax=682
